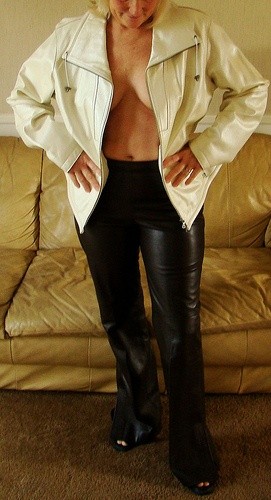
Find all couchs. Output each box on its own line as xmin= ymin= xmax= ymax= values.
xmin=0 ymin=131 xmax=270 ymax=404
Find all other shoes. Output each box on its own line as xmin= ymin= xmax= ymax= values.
xmin=172 ymin=458 xmax=217 ymax=495
xmin=110 ymin=427 xmax=156 ymax=452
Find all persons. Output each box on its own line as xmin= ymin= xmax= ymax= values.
xmin=5 ymin=0 xmax=270 ymax=497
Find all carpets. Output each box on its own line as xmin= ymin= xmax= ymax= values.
xmin=0 ymin=384 xmax=271 ymax=499
